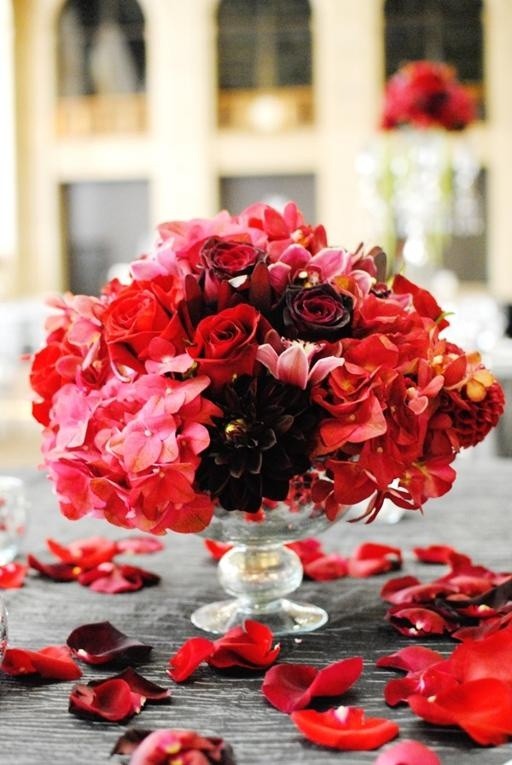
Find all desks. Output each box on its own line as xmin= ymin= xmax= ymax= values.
xmin=0 ymin=460 xmax=512 ymax=764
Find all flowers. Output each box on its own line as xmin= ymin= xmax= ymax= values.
xmin=29 ymin=201 xmax=501 ymax=535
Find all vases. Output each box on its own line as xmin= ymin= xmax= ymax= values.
xmin=191 ymin=491 xmax=331 ymax=635
